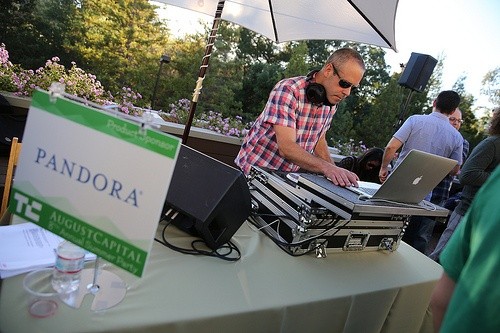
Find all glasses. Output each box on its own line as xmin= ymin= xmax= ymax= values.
xmin=332 ymin=64 xmax=358 ymax=94
xmin=449 ymin=117 xmax=464 ymax=124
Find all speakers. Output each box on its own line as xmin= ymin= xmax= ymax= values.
xmin=160 ymin=143 xmax=252 ymax=250
xmin=397 ymin=52 xmax=438 ymax=93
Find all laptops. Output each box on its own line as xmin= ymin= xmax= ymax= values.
xmin=326 ymin=148 xmax=459 ymax=205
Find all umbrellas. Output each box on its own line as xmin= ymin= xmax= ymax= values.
xmin=151 ymin=0 xmax=400 ymax=144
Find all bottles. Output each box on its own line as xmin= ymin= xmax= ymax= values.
xmin=50 ymin=240 xmax=86 ymax=294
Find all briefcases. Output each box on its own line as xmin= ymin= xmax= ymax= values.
xmin=247 ymin=166 xmax=410 ymax=258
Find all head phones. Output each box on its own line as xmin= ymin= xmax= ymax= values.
xmin=305 ymin=69 xmax=336 ymax=107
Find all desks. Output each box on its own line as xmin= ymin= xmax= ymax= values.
xmin=0 ymin=221 xmax=445 ymax=333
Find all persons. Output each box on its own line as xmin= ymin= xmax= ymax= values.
xmin=429 ymin=107 xmax=500 ymax=263
xmin=430 ymin=164 xmax=500 ymax=333
xmin=234 ymin=48 xmax=367 ymax=188
xmin=402 ymin=108 xmax=469 ymax=253
xmin=338 ymin=148 xmax=385 ymax=183
xmin=379 ymin=90 xmax=464 ymax=202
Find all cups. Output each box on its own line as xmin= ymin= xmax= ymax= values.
xmin=23 ymin=268 xmax=73 ymax=317
